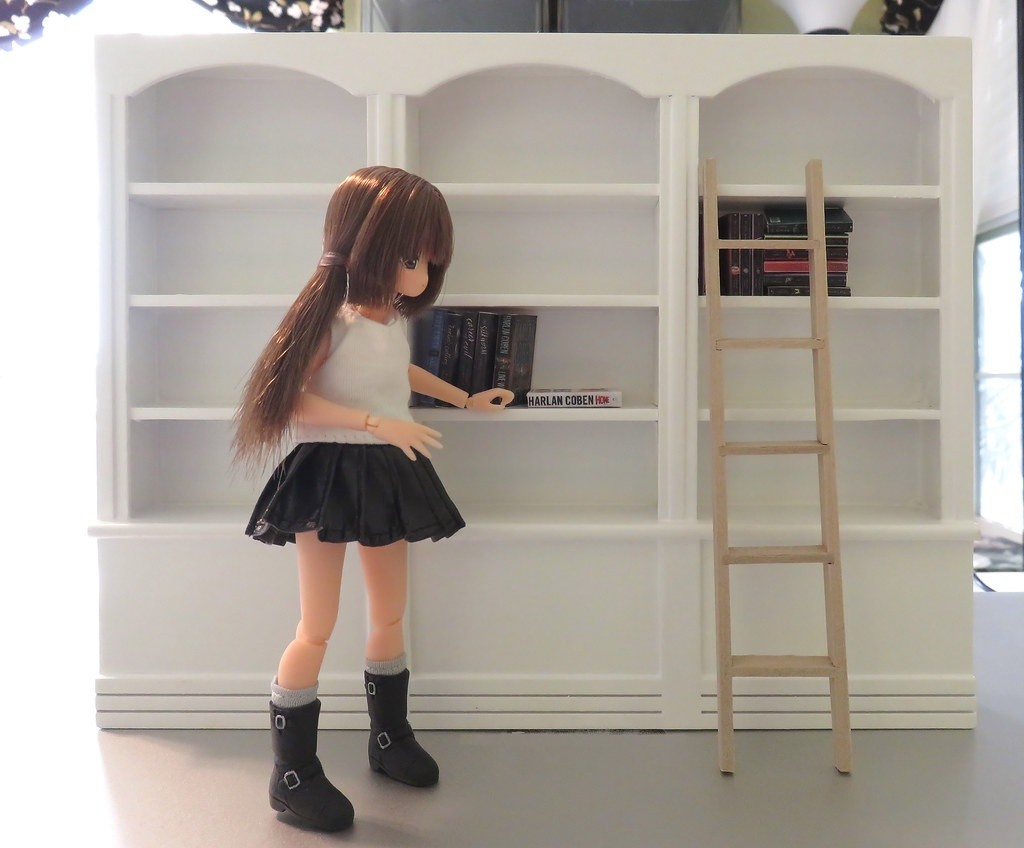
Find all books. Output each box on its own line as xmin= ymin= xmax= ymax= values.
xmin=420 ymin=310 xmax=623 ymax=408
xmin=698 ymin=207 xmax=853 ymax=298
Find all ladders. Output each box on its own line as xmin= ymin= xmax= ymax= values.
xmin=696 ymin=148 xmax=858 ymax=778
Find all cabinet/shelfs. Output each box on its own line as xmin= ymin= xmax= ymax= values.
xmin=89 ymin=33 xmax=977 ymax=731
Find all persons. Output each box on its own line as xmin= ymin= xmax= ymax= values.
xmin=224 ymin=166 xmax=514 ymax=831
